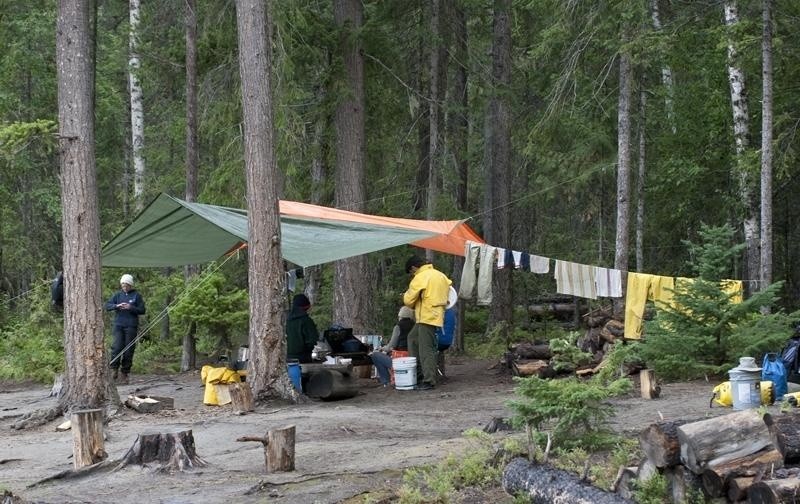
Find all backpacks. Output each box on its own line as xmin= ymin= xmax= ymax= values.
xmin=323 ymin=328 xmax=373 ymax=360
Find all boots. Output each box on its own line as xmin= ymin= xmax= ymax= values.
xmin=112 ymin=368 xmax=129 ymax=384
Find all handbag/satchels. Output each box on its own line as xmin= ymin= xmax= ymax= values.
xmin=762 ymin=353 xmax=788 ymax=400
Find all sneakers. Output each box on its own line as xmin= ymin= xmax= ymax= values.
xmin=415 ymin=382 xmax=432 ymax=390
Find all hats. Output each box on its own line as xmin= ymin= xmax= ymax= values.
xmin=406 ymin=256 xmax=420 ymax=273
xmin=120 ymin=274 xmax=134 ymax=286
xmin=293 ymin=294 xmax=310 ymax=310
xmin=398 ymin=306 xmax=413 ymax=318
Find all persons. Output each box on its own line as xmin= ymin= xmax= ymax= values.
xmin=403 ymin=255 xmax=452 ymax=390
xmin=372 ymin=306 xmax=415 ymax=388
xmin=286 ymin=294 xmax=319 ymax=363
xmin=104 ymin=274 xmax=145 ymax=379
xmin=434 ymin=308 xmax=455 ymax=350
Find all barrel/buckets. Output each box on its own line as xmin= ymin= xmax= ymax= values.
xmin=391 ymin=356 xmax=418 ymax=392
xmin=389 ymin=349 xmax=408 ymax=385
xmin=286 ymin=358 xmax=304 ymax=394
xmin=728 ymin=370 xmax=763 ymax=413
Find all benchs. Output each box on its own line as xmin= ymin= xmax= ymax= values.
xmin=300 ymin=362 xmax=350 ymax=374
